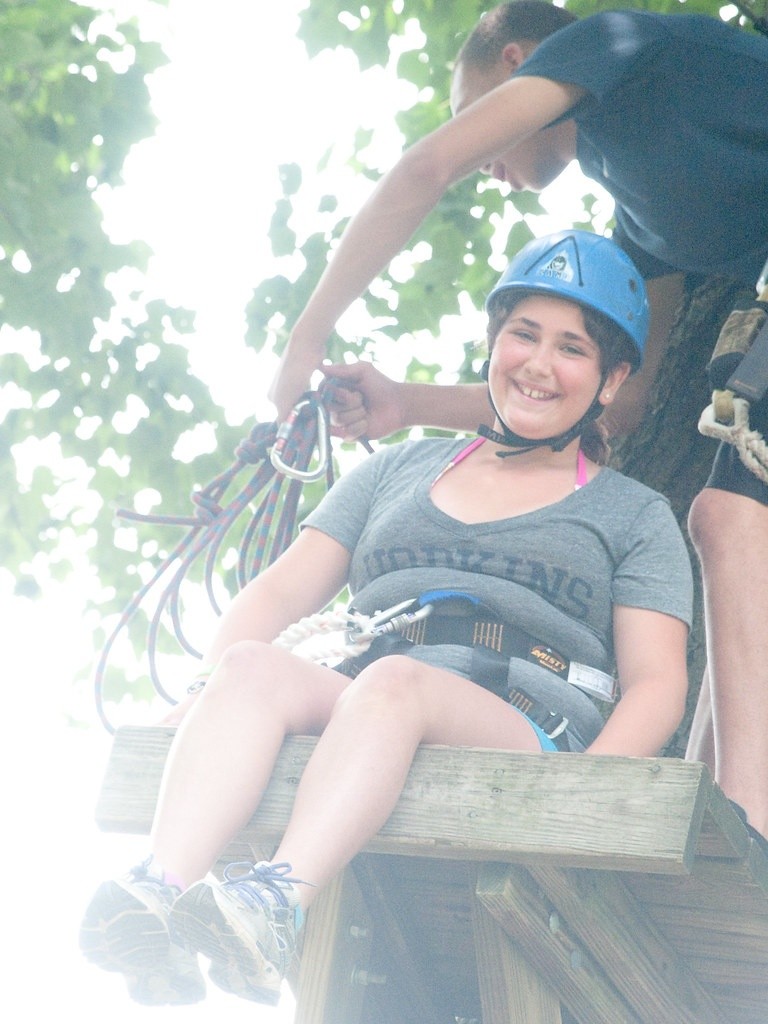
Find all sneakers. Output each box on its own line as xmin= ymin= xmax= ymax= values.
xmin=82 ymin=862 xmax=205 ymax=1004
xmin=168 ymin=861 xmax=317 ymax=1007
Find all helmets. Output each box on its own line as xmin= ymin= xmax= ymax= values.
xmin=484 ymin=229 xmax=651 ymax=375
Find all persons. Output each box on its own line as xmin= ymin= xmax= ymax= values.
xmin=68 ymin=230 xmax=692 ymax=1005
xmin=269 ymin=1 xmax=768 ymax=846
